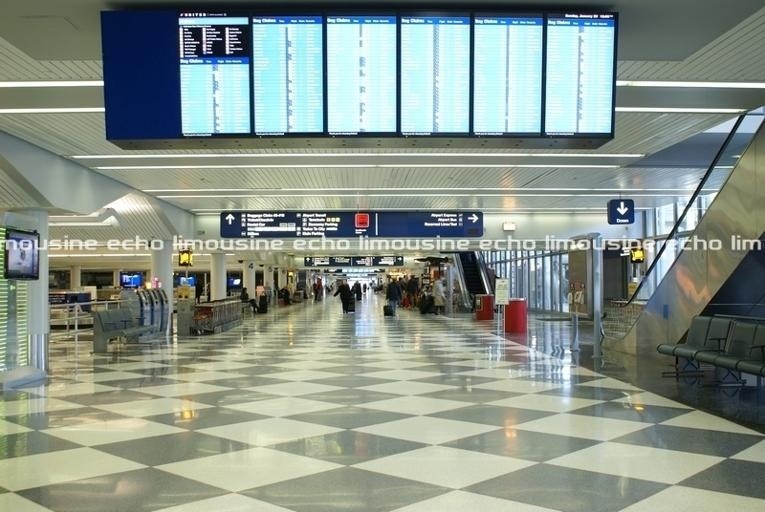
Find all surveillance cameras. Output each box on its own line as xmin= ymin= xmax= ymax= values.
xmin=239 ymin=260 xmax=243 ymax=263
xmin=260 ymin=264 xmax=264 ymax=267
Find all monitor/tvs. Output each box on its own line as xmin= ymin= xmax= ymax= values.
xmin=227 ymin=276 xmax=242 ymax=288
xmin=176 ymin=276 xmax=195 ymax=286
xmin=120 ymin=273 xmax=142 ymax=288
xmin=4 ymin=228 xmax=40 ymax=281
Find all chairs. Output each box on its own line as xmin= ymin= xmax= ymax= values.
xmin=655 ymin=313 xmax=765 ymax=388
xmin=90 ymin=306 xmax=154 ymax=354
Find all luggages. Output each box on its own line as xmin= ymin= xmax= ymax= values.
xmin=383 ymin=298 xmax=394 ymax=316
xmin=348 ymin=294 xmax=356 ymax=312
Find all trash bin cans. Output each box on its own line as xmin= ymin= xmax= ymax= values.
xmin=505 ymin=298 xmax=527 ymax=333
xmin=475 ymin=294 xmax=495 ymax=320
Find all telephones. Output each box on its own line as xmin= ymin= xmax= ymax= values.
xmin=574 ymin=290 xmax=584 ymax=305
xmin=567 ymin=293 xmax=573 ymax=304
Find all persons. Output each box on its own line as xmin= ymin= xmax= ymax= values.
xmin=86 ymin=273 xmax=102 ymax=289
xmin=240 ymin=275 xmax=460 ymax=320
xmin=194 ymin=279 xmax=210 ymax=304
